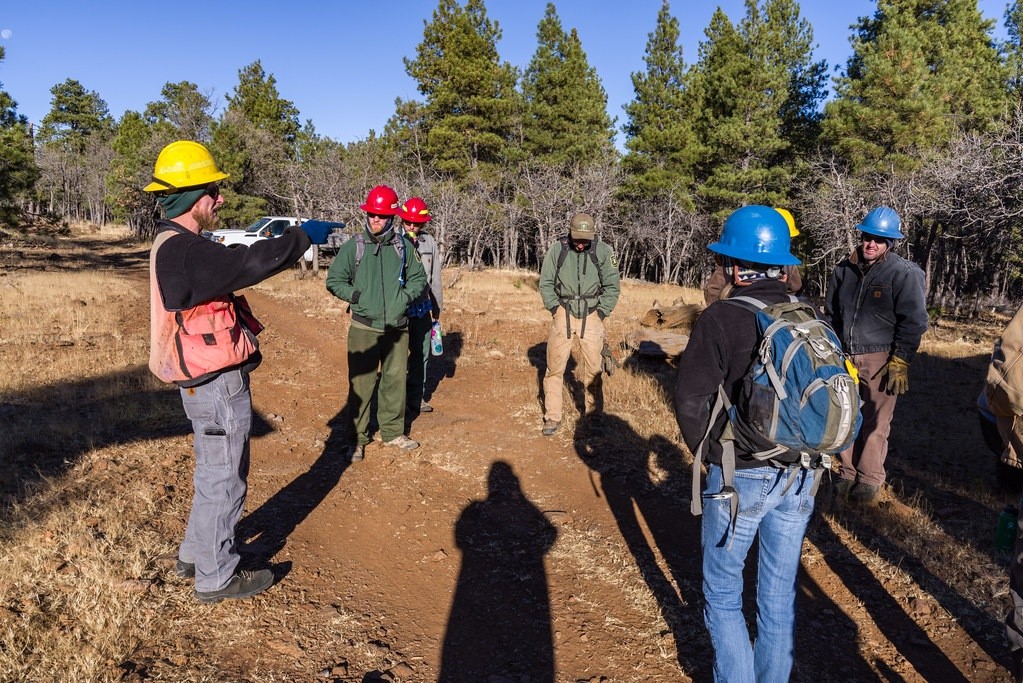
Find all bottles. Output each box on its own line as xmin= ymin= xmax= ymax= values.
xmin=430 ymin=321 xmax=443 ymax=356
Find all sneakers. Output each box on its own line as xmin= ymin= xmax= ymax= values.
xmin=543 ymin=419 xmax=561 ymax=436
xmin=194 ymin=569 xmax=275 ymax=604
xmin=346 ymin=445 xmax=364 ymax=461
xmin=835 ymin=477 xmax=855 ymax=497
xmin=384 ymin=434 xmax=419 ymax=450
xmin=851 ymin=481 xmax=878 ymax=503
xmin=411 ymin=401 xmax=433 ymax=412
xmin=175 ymin=559 xmax=195 ymax=579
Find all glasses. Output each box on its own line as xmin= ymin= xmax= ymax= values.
xmin=713 ymin=255 xmax=722 ymax=266
xmin=572 ymin=241 xmax=591 ymax=246
xmin=860 ymin=233 xmax=887 ymax=243
xmin=367 ymin=213 xmax=389 ymax=218
xmin=402 ymin=221 xmax=422 ymax=227
xmin=203 ymin=186 xmax=219 ymax=199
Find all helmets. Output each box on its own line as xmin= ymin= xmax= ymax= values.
xmin=398 ymin=197 xmax=431 ymax=222
xmin=707 ymin=205 xmax=802 ymax=265
xmin=774 ymin=207 xmax=800 ymax=237
xmin=143 ymin=140 xmax=230 ymax=191
xmin=360 ymin=185 xmax=399 ymax=215
xmin=853 ymin=206 xmax=904 ymax=238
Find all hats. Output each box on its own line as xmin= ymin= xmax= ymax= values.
xmin=157 ymin=189 xmax=205 ymax=218
xmin=570 ymin=213 xmax=595 ymax=240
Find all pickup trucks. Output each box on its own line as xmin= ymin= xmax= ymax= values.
xmin=202 ymin=216 xmax=352 ymax=269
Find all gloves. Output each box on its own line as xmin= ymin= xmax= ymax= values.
xmin=873 ymin=355 xmax=910 ymax=394
xmin=300 ymin=219 xmax=346 ymax=244
xmin=550 ymin=305 xmax=559 ymax=317
xmin=598 ymin=309 xmax=605 ymax=321
xmin=352 ymin=291 xmax=361 ymax=304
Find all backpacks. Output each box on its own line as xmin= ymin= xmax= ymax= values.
xmin=690 ymin=296 xmax=864 ymax=513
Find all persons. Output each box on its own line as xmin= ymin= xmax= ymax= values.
xmin=977 ymin=302 xmax=1023 ymax=683
xmin=540 ymin=213 xmax=620 ymax=434
xmin=326 ymin=186 xmax=428 ymax=462
xmin=824 ymin=207 xmax=928 ymax=500
xmin=143 ymin=140 xmax=346 ymax=602
xmin=675 ymin=204 xmax=821 ymax=683
xmin=395 ymin=197 xmax=443 ymax=411
xmin=704 ymin=209 xmax=803 ymax=306
